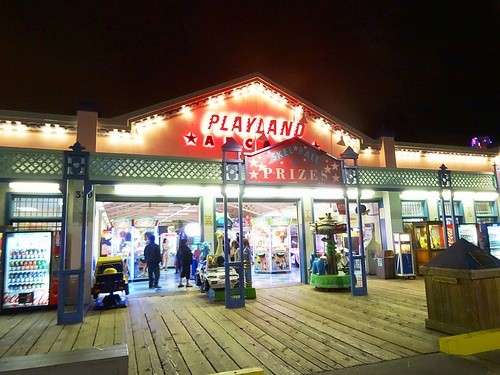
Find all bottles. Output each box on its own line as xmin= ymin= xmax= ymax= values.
xmin=9 ymin=249 xmax=47 ymax=289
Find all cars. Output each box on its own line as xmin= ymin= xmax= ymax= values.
xmin=195 ymin=259 xmax=240 ymax=292
xmin=90 ymin=255 xmax=130 ymax=299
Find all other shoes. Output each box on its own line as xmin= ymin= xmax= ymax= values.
xmin=149 ymin=285 xmax=154 ymax=288
xmin=186 ymin=284 xmax=193 ymax=287
xmin=153 ymin=285 xmax=161 ymax=288
xmin=178 ymin=284 xmax=184 ymax=287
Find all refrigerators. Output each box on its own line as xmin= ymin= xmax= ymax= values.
xmin=0 ymin=230 xmax=53 ymax=311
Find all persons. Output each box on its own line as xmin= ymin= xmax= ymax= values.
xmin=176 ymin=239 xmax=193 ymax=287
xmin=174 ymin=254 xmax=181 ymax=274
xmin=120 ymin=239 xmax=127 ymax=253
xmin=160 ymin=238 xmax=170 ymax=271
xmin=236 ymin=233 xmax=239 ymax=242
xmin=194 ymin=243 xmax=202 ymax=267
xmin=143 ymin=235 xmax=163 ymax=289
xmin=243 ymin=238 xmax=252 ymax=287
xmin=232 ymin=241 xmax=240 ymax=261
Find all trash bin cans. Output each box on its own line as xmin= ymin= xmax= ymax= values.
xmin=373 ymin=250 xmax=395 ymax=279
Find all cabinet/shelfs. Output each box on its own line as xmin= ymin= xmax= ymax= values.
xmin=393 ymin=231 xmax=414 ymax=279
xmin=413 ymin=221 xmax=445 ymax=266
